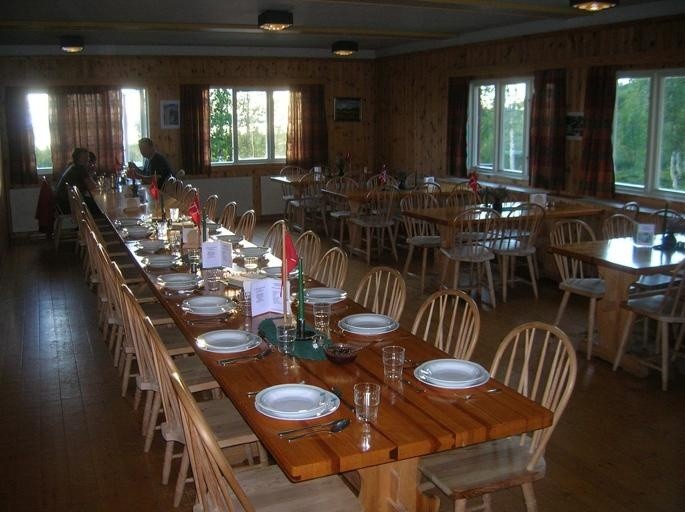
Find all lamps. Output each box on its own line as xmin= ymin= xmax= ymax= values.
xmin=256 ymin=10 xmax=295 ymax=33
xmin=60 ymin=35 xmax=85 ymax=52
xmin=569 ymin=1 xmax=618 ymax=14
xmin=331 ymin=40 xmax=361 ymax=57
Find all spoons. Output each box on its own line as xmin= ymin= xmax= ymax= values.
xmin=276 ymin=415 xmax=353 ymax=442
xmin=455 ymin=386 xmax=505 ymax=402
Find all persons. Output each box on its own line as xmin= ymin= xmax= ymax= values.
xmin=56 ymin=151 xmax=97 ymax=187
xmin=127 ymin=139 xmax=176 ymax=189
xmin=55 ymin=147 xmax=97 ymax=214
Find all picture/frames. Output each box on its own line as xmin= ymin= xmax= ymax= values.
xmin=158 ymin=98 xmax=181 ymax=129
xmin=562 ymin=111 xmax=583 ymax=140
xmin=333 ymin=95 xmax=362 ymax=124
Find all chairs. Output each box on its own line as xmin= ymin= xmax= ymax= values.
xmin=84 ymin=218 xmax=577 ymax=511
xmin=271 ymin=166 xmax=578 ymax=219
xmin=577 ymin=202 xmax=685 ymax=391
xmin=41 ymin=169 xmax=257 ymax=291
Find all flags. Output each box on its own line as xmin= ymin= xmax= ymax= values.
xmin=188 ymin=187 xmax=201 ymax=236
xmin=469 ymin=168 xmax=478 ymax=192
xmin=149 ymin=172 xmax=160 ymax=207
xmin=281 ymin=230 xmax=299 ymax=285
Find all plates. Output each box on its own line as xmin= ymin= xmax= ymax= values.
xmin=110 ymin=217 xmax=491 ymax=422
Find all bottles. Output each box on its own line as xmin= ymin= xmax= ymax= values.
xmin=109 ymin=171 xmax=118 ymax=189
